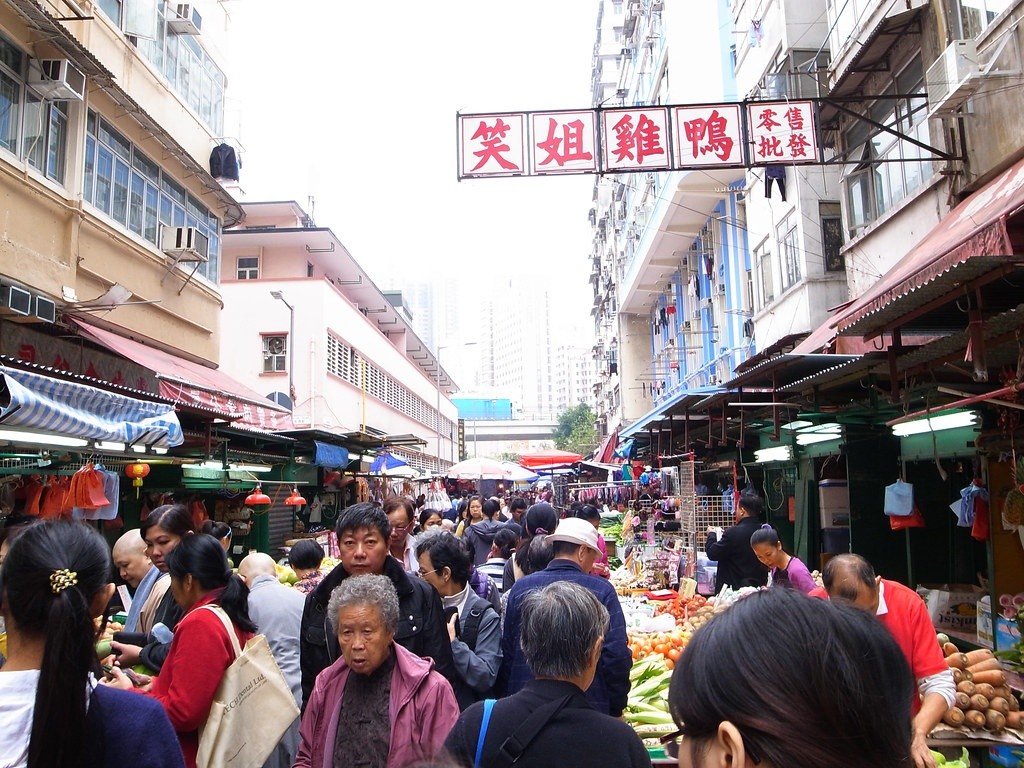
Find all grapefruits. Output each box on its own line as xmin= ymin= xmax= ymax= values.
xmin=275 ymin=565 xmax=300 ymax=588
xmin=320 ymin=557 xmax=342 ymax=567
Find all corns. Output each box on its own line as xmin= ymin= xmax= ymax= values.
xmin=625 ymin=652 xmax=684 ymax=747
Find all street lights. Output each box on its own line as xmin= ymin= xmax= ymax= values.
xmin=271 ymin=290 xmax=293 ymax=413
xmin=437 ymin=342 xmax=478 ymax=476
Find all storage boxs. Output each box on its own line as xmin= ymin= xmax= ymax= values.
xmin=915 ymin=583 xmax=986 ymax=631
xmin=989 ymin=745 xmax=1024 ymax=768
xmin=995 ymin=619 xmax=1024 ymax=679
xmin=976 ymin=600 xmax=994 ymax=650
xmin=678 ymin=578 xmax=697 ymax=600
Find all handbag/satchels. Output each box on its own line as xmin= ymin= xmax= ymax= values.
xmin=176 ymin=603 xmax=301 ymax=768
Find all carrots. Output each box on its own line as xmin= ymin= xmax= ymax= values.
xmin=938 ymin=641 xmax=1024 ymax=730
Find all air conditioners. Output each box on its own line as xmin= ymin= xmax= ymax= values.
xmin=926 ymin=39 xmax=980 ymax=112
xmin=27 ymin=58 xmax=86 ymax=101
xmin=161 ymin=225 xmax=210 ymax=263
xmin=166 ymin=4 xmax=202 ymax=35
xmin=692 ymin=309 xmax=700 ymax=320
xmin=700 ymin=297 xmax=712 ymax=310
xmin=681 ymin=320 xmax=691 ymax=330
xmin=709 ymin=374 xmax=717 ymax=384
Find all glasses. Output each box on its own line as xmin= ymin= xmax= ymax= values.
xmin=394 ymin=521 xmax=415 ymax=534
xmin=659 ymin=727 xmax=689 ymax=761
xmin=417 ymin=569 xmax=436 ymax=577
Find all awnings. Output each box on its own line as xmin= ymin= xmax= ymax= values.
xmin=0 ymin=352 xmax=245 ymax=447
xmin=72 ymin=316 xmax=295 ymax=432
xmin=630 ymin=392 xmax=806 ymax=456
xmin=719 ymin=303 xmax=938 ymax=398
xmin=827 ymin=158 xmax=1024 ymax=337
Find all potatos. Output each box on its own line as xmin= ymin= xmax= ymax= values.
xmin=93 ymin=615 xmax=125 ymax=640
xmin=654 ymin=595 xmax=724 ymax=633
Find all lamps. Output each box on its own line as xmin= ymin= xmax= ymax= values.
xmin=324 ymin=470 xmax=354 ymax=487
xmin=714 ymin=284 xmax=725 ymax=296
xmin=753 ymin=444 xmax=794 ymax=463
xmin=280 ymin=484 xmax=307 ymax=505
xmin=892 ymin=410 xmax=982 ymax=436
xmin=239 ymin=465 xmax=272 ymax=506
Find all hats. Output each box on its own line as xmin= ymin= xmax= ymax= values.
xmin=545 ymin=517 xmax=603 ymax=557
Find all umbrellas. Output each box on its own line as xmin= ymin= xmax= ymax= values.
xmin=447 ymin=448 xmax=584 ymax=495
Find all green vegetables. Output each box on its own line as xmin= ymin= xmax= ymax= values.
xmin=599 ymin=513 xmax=624 ymax=570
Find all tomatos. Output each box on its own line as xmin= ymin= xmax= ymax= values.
xmin=626 ymin=634 xmax=686 ymax=670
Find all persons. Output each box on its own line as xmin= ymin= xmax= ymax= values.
xmin=290 ymin=573 xmax=461 ymax=768
xmin=410 ymin=486 xmax=610 ymax=601
xmin=300 ymin=501 xmax=459 ymax=724
xmin=382 ymin=496 xmax=422 ymax=576
xmin=437 ymin=579 xmax=652 ymax=768
xmin=0 ymin=516 xmax=183 ymax=768
xmin=289 ymin=539 xmax=329 ymax=595
xmin=236 ymin=553 xmax=307 ymax=768
xmin=98 ymin=505 xmax=254 ymax=768
xmin=805 ymin=553 xmax=956 ymax=768
xmin=704 ymin=492 xmax=770 ymax=596
xmin=667 ymin=586 xmax=918 ymax=768
xmin=502 ymin=516 xmax=633 ymax=718
xmin=414 ymin=530 xmax=501 ymax=715
xmin=751 ymin=523 xmax=817 ymax=594
